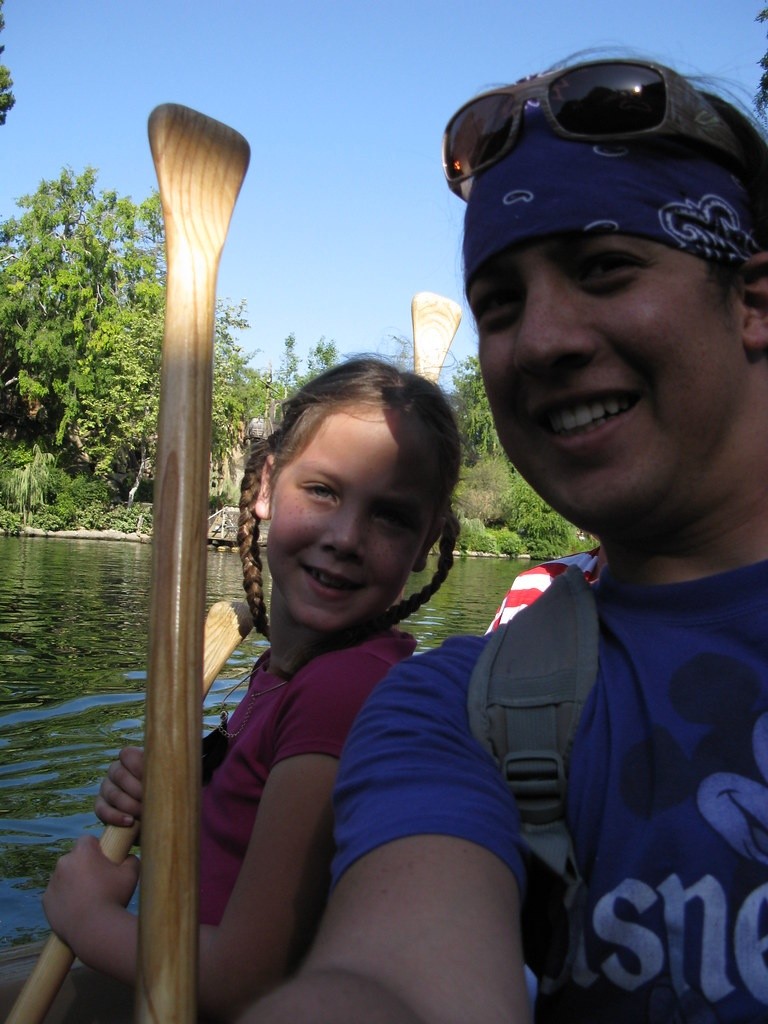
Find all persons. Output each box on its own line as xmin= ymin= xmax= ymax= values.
xmin=241 ymin=56 xmax=768 ymax=1022
xmin=39 ymin=355 xmax=461 ymax=1020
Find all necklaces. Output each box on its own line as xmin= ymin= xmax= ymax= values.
xmin=217 ymin=657 xmax=288 ymax=739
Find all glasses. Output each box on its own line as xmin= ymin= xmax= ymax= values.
xmin=439 ymin=58 xmax=749 ymax=204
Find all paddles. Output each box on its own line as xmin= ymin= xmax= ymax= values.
xmin=6 ymin=594 xmax=255 ymax=1024
xmin=135 ymin=101 xmax=253 ymax=1021
